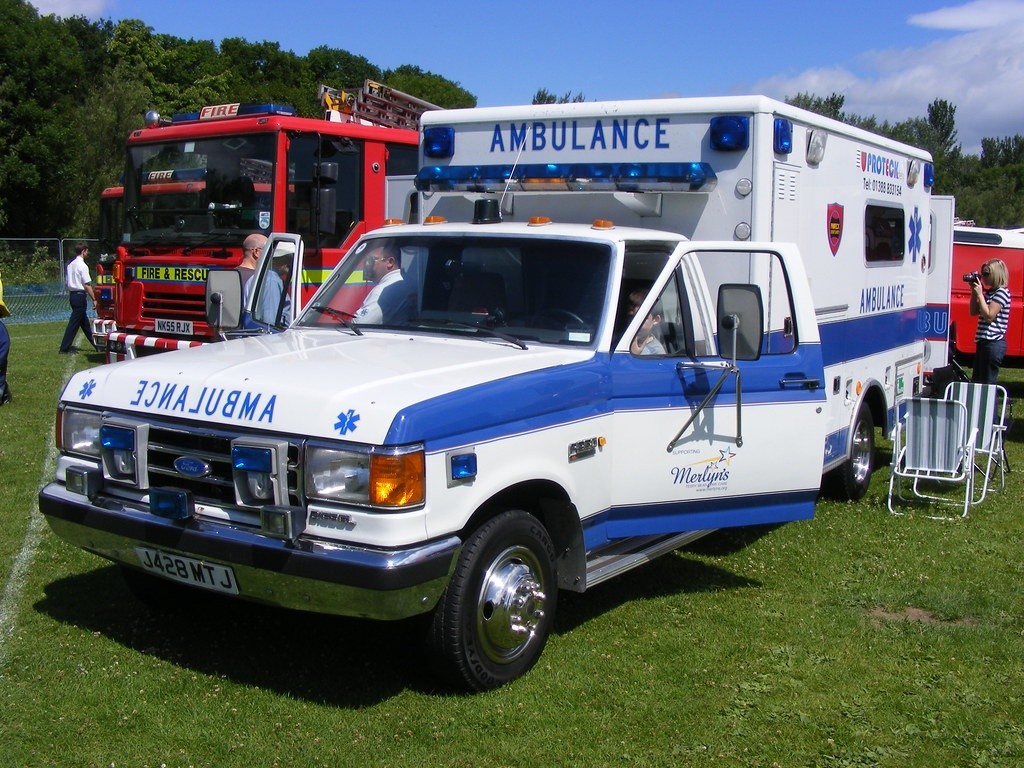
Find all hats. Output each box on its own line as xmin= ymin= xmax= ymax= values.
xmin=0 ymin=281 xmax=12 ymax=317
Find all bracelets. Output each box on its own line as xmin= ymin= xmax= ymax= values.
xmin=92 ymin=299 xmax=97 ymax=301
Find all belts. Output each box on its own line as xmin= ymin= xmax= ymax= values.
xmin=71 ymin=291 xmax=85 ymax=295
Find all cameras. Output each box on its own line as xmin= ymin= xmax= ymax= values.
xmin=963 ymin=273 xmax=981 ymax=284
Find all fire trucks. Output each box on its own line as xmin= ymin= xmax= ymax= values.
xmin=92 ymin=156 xmax=294 ymax=350
xmin=108 ymin=81 xmax=454 ymax=370
xmin=948 ymin=219 xmax=1023 ymax=360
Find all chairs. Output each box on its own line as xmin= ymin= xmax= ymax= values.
xmin=426 ymin=264 xmax=626 ymax=340
xmin=914 ymin=382 xmax=1007 ymax=505
xmin=888 ymin=396 xmax=979 ymax=521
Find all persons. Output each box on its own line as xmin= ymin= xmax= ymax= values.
xmin=59 ymin=242 xmax=98 ymax=354
xmin=617 ymin=289 xmax=666 ymax=355
xmin=970 ymin=258 xmax=1011 ymax=439
xmin=233 ymin=233 xmax=297 ymax=328
xmin=350 ymin=247 xmax=404 ymax=324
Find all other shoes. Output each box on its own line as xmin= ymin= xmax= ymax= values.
xmin=96 ymin=348 xmax=105 ymax=352
xmin=59 ymin=349 xmax=76 ymax=354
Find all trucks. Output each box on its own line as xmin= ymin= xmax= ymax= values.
xmin=35 ymin=95 xmax=958 ymax=691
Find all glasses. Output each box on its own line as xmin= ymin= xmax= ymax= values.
xmin=368 ymin=257 xmax=386 ymax=265
